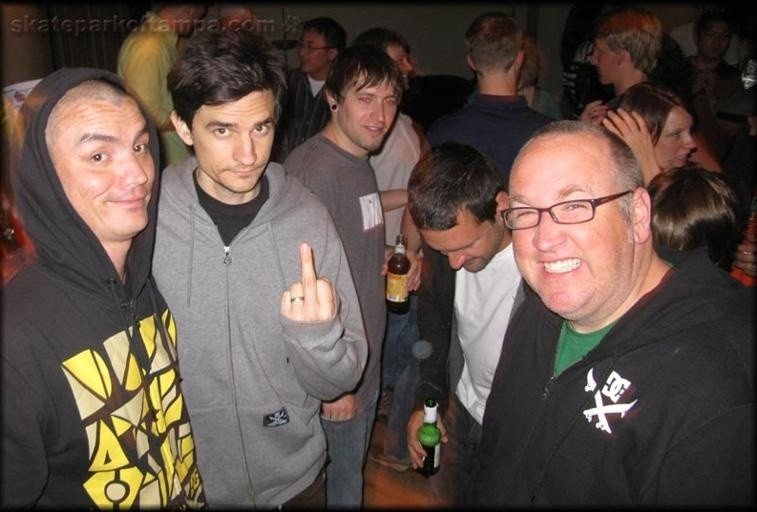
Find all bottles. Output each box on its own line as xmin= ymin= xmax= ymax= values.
xmin=413 ymin=398 xmax=442 ymax=478
xmin=384 ymin=233 xmax=411 ymax=316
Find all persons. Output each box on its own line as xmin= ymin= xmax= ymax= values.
xmin=115 ymin=1 xmax=756 ymax=511
xmin=0 ymin=64 xmax=209 ymax=511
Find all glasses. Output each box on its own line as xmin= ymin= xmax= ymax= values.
xmin=499 ymin=190 xmax=635 ymax=231
xmin=298 ymin=40 xmax=330 ymax=52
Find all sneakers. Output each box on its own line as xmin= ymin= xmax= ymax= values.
xmin=376 ymin=392 xmax=392 ymax=424
xmin=368 ymin=440 xmax=410 ymax=472
